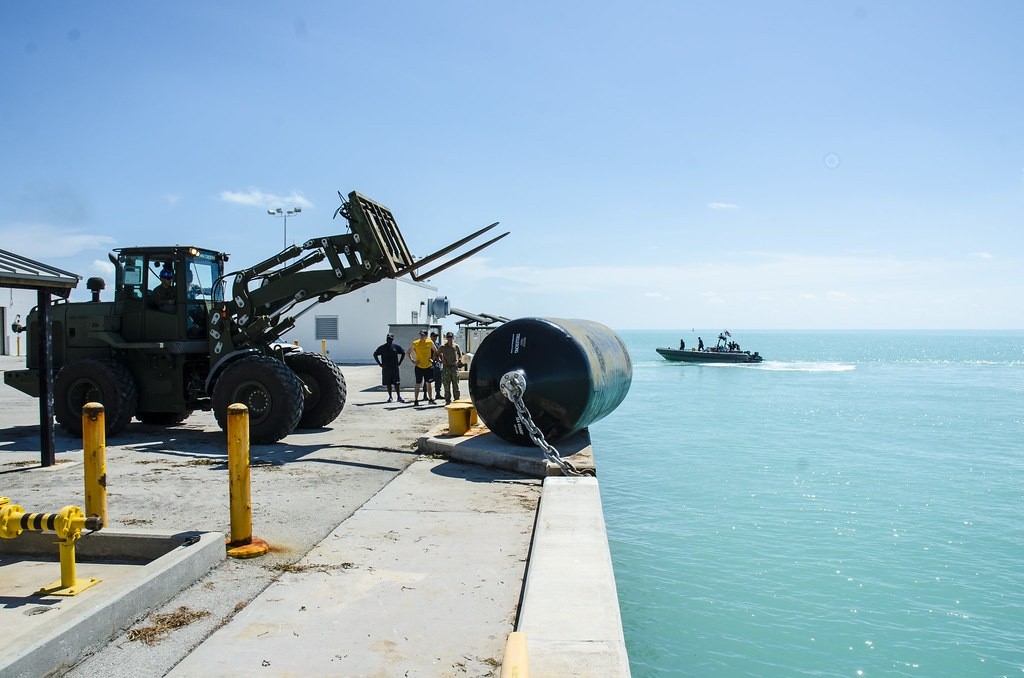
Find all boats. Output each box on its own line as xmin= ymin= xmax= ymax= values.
xmin=656 ymin=332 xmax=765 ymax=365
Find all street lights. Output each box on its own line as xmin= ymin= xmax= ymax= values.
xmin=266 ymin=204 xmax=301 ymax=273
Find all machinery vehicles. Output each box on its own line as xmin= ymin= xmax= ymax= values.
xmin=13 ymin=190 xmax=510 ymax=444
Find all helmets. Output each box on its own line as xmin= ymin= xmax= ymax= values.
xmin=160 ymin=269 xmax=175 ymax=279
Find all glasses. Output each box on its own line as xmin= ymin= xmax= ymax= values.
xmin=447 ymin=335 xmax=453 ymax=339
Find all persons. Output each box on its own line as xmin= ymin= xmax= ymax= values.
xmin=680 ymin=339 xmax=685 ymax=350
xmin=698 ymin=337 xmax=704 ymax=351
xmin=423 ymin=332 xmax=462 ymax=405
xmin=727 ymin=341 xmax=740 ymax=352
xmin=407 ymin=330 xmax=438 ymax=406
xmin=373 ymin=333 xmax=406 ymax=403
xmin=152 ymin=268 xmax=194 ymax=311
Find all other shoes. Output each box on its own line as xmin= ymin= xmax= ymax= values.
xmin=429 ymin=400 xmax=436 ymax=404
xmin=397 ymin=397 xmax=404 ymax=402
xmin=446 ymin=400 xmax=451 ymax=405
xmin=436 ymin=395 xmax=445 ymax=399
xmin=424 ymin=397 xmax=429 ymax=401
xmin=414 ymin=401 xmax=418 ymax=405
xmin=387 ymin=398 xmax=393 ymax=402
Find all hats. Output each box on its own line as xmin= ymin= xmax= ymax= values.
xmin=419 ymin=330 xmax=428 ymax=336
xmin=387 ymin=333 xmax=394 ymax=340
xmin=430 ymin=332 xmax=440 ymax=337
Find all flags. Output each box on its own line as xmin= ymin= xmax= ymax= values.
xmin=725 ymin=330 xmax=732 ymax=337
